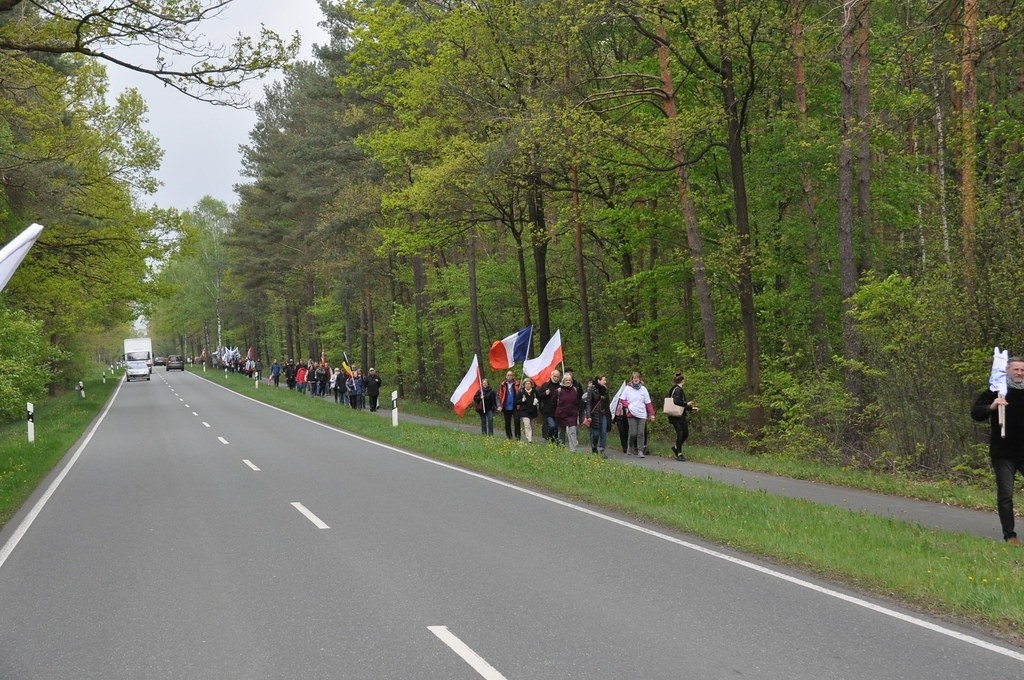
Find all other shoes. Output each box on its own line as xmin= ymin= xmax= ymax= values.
xmin=592 ymin=447 xmax=598 ymax=454
xmin=633 ymin=448 xmax=638 ymax=455
xmin=623 ymin=446 xmax=628 ymax=453
xmin=600 ymin=450 xmax=608 ymax=459
xmin=548 ymin=435 xmax=557 ymax=444
xmin=671 ymin=446 xmax=679 ymax=457
xmin=1007 ymin=537 xmax=1019 ymax=545
xmin=677 ymin=455 xmax=685 ymax=461
xmin=643 ymin=447 xmax=649 ymax=455
xmin=638 ymin=450 xmax=645 ymax=458
xmin=627 ymin=446 xmax=633 ymax=455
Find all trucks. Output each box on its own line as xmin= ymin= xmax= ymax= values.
xmin=122 ymin=337 xmax=155 ymax=374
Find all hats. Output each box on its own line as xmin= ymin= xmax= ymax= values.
xmin=522 ymin=378 xmax=534 ymax=387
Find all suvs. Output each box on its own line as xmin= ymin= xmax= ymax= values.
xmin=165 ymin=354 xmax=184 ymax=372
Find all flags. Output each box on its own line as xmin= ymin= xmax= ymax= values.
xmin=523 ymin=329 xmax=564 ymax=389
xmin=489 ymin=326 xmax=533 ymax=372
xmin=341 ymin=353 xmax=352 ymax=379
xmin=450 ymin=355 xmax=480 ymax=418
xmin=989 ymin=350 xmax=1010 ymax=393
xmin=247 ymin=347 xmax=254 ymax=360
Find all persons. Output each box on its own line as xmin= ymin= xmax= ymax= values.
xmin=270 ymin=359 xmax=382 ymax=412
xmin=970 ymin=357 xmax=1023 ymax=547
xmin=670 ymin=374 xmax=698 ymax=461
xmin=475 ymin=372 xmax=655 ymax=459
xmin=202 ymin=354 xmax=263 ymax=380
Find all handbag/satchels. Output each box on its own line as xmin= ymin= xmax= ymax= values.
xmin=663 ymin=387 xmax=685 ymax=417
xmin=616 ymin=403 xmax=623 ymax=416
xmin=476 ymin=401 xmax=483 ymax=412
xmin=583 ymin=417 xmax=590 ymax=427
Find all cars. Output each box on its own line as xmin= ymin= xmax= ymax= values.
xmin=154 ymin=357 xmax=166 ymax=367
xmin=125 ymin=361 xmax=152 ymax=382
